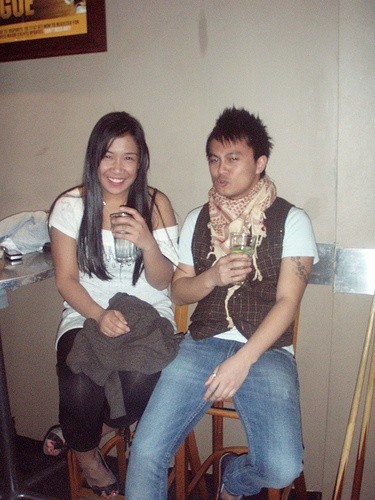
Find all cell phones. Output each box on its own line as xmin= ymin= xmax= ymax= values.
xmin=3 ymin=247 xmax=22 ymax=261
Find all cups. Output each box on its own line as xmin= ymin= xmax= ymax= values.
xmin=111 ymin=213 xmax=138 ymax=262
xmin=230 ymin=232 xmax=258 ymax=285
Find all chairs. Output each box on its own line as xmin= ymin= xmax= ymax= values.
xmin=67 ymin=304 xmax=305 ymax=500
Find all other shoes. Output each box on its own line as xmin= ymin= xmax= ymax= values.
xmin=216 ymin=452 xmax=244 ymax=500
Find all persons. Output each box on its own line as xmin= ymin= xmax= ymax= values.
xmin=125 ymin=109 xmax=320 ymax=500
xmin=42 ymin=112 xmax=180 ymax=500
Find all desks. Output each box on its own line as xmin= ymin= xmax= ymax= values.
xmin=0 ymin=243 xmax=65 ymax=500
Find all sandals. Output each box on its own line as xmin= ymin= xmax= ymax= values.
xmin=74 ymin=448 xmax=120 ymax=498
xmin=42 ymin=424 xmax=68 ymax=457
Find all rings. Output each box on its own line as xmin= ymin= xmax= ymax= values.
xmin=212 ymin=373 xmax=216 ymax=377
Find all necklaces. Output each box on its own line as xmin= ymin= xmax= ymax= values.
xmin=102 ymin=199 xmax=128 ymax=214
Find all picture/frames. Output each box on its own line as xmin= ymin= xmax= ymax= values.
xmin=0 ymin=0 xmax=108 ymax=63
